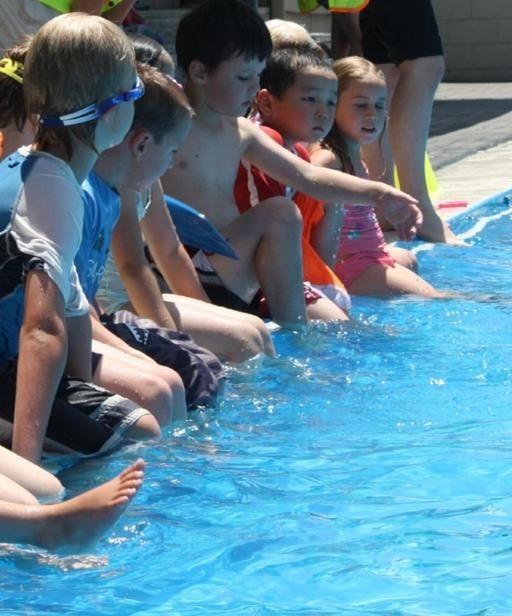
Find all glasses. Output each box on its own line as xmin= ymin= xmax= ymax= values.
xmin=37 ymin=77 xmax=145 ymax=128
